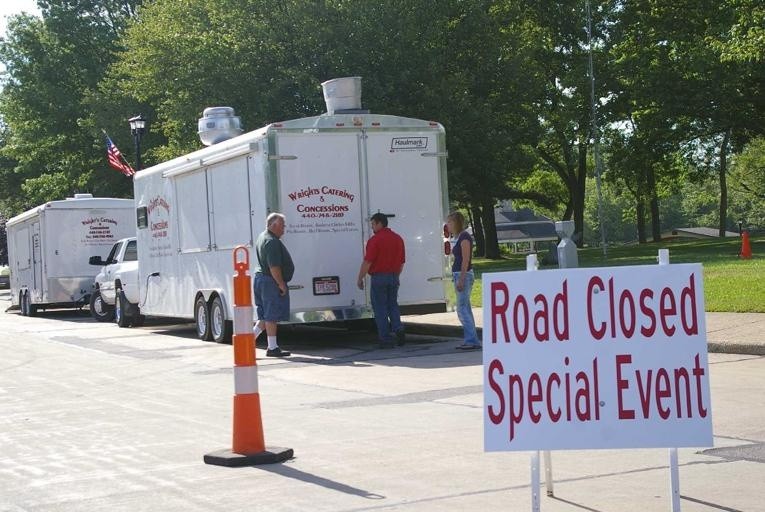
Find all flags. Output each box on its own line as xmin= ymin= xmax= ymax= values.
xmin=104 ymin=133 xmax=135 ymax=177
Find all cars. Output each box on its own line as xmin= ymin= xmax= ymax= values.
xmin=0 ymin=275 xmax=10 ymax=290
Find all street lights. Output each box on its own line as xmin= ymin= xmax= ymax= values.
xmin=127 ymin=112 xmax=146 ymax=171
xmin=738 ymin=218 xmax=742 ymax=236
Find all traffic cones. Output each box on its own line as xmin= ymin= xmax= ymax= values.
xmin=740 ymin=231 xmax=753 ymax=258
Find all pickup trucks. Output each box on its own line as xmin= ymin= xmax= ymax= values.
xmin=89 ymin=236 xmax=145 ymax=328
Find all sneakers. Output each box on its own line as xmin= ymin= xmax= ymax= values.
xmin=456 ymin=344 xmax=481 ymax=350
xmin=372 ymin=332 xmax=407 ymax=350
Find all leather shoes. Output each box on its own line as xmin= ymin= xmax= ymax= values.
xmin=266 ymin=346 xmax=291 ymax=357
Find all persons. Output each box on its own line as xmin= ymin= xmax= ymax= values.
xmin=252 ymin=212 xmax=294 ymax=357
xmin=445 ymin=212 xmax=481 ymax=350
xmin=357 ymin=213 xmax=406 ymax=344
